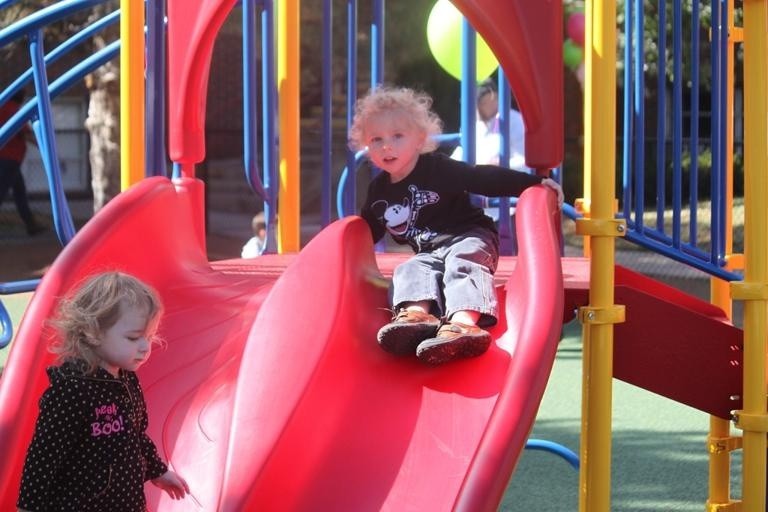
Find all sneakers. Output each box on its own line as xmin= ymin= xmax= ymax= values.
xmin=417 ymin=320 xmax=491 ymax=366
xmin=26 ymin=221 xmax=48 ymax=235
xmin=378 ymin=308 xmax=439 ymax=356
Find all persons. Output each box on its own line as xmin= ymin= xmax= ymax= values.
xmin=347 ymin=84 xmax=565 ymax=363
xmin=1 ymin=89 xmax=46 ymax=235
xmin=15 ymin=272 xmax=190 ymax=510
xmin=447 ymin=79 xmax=533 ymax=173
xmin=240 ymin=210 xmax=268 ymax=258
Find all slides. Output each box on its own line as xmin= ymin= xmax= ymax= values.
xmin=1 ymin=176 xmax=564 ymax=512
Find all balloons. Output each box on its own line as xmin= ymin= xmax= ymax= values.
xmin=563 ymin=12 xmax=584 ymax=86
xmin=426 ymin=0 xmax=499 ymax=83
